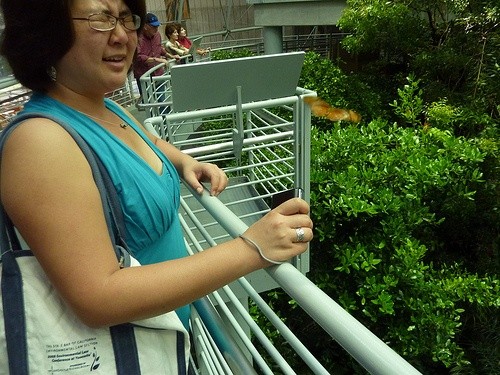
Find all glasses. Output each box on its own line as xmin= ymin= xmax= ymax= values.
xmin=71 ymin=13 xmax=142 ymax=31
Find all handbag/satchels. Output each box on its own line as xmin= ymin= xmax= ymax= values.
xmin=0 ymin=245 xmax=191 ymax=375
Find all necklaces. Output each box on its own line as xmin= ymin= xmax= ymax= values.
xmin=78 ymin=110 xmax=129 ymax=128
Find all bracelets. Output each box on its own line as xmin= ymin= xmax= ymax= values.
xmin=154 ymin=58 xmax=157 ymax=63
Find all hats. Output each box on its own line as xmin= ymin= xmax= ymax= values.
xmin=145 ymin=13 xmax=161 ymax=26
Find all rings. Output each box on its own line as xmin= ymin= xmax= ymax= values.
xmin=296 ymin=227 xmax=304 ymax=242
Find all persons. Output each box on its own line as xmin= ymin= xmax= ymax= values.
xmin=132 ymin=12 xmax=208 ymax=141
xmin=0 ymin=0 xmax=313 ymax=354
